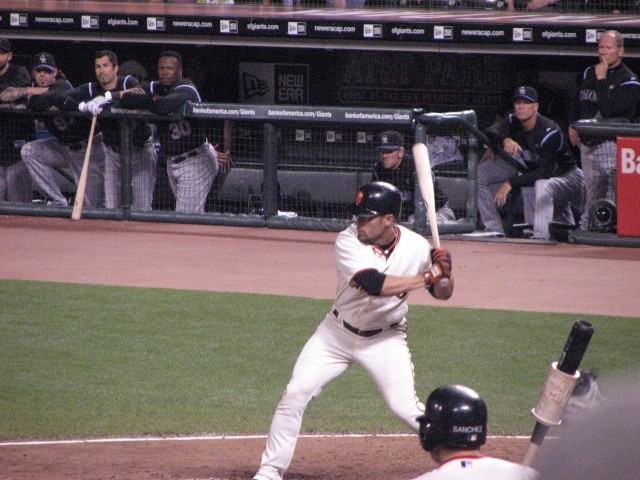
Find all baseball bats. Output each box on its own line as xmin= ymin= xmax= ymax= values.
xmin=412 ymin=143 xmax=440 ymax=249
xmin=523 ymin=320 xmax=594 ymax=469
xmin=70 ymin=116 xmax=97 ymax=220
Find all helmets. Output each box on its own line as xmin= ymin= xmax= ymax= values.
xmin=416 ymin=384 xmax=488 ymax=452
xmin=349 ymin=181 xmax=402 ymax=219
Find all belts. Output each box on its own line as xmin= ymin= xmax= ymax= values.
xmin=332 ymin=309 xmax=397 ymax=336
xmin=167 ymin=151 xmax=198 ymax=163
xmin=68 ymin=141 xmax=93 ymax=150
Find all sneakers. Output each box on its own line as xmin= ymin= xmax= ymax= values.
xmin=252 ymin=466 xmax=282 ymax=480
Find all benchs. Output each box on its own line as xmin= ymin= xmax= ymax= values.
xmin=146 ymin=144 xmax=512 ymax=218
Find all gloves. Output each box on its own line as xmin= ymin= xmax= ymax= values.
xmin=88 ymin=90 xmax=113 ymax=116
xmin=422 ymin=248 xmax=451 ymax=285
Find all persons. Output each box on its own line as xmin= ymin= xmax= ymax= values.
xmin=252 ymin=181 xmax=454 ymax=480
xmin=0 ymin=52 xmax=106 ymax=208
xmin=54 ymin=50 xmax=158 ymax=211
xmin=212 ymin=119 xmax=235 ymax=174
xmin=326 ymin=0 xmax=366 ymax=9
xmin=260 ymin=0 xmax=272 ymax=7
xmin=0 ymin=37 xmax=36 ymax=203
xmin=480 ymin=112 xmax=504 ymax=165
xmin=119 ymin=51 xmax=219 ymax=213
xmin=372 ymin=130 xmax=456 ymax=225
xmin=476 ymin=86 xmax=585 ymax=242
xmin=568 ymin=29 xmax=640 ymax=232
xmin=407 ymin=384 xmax=541 ymax=480
xmin=505 ymin=0 xmax=560 ymax=13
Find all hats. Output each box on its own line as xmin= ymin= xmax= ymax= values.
xmin=2 ymin=33 xmax=12 ymax=55
xmin=375 ymin=130 xmax=403 ymax=151
xmin=512 ymin=86 xmax=538 ymax=102
xmin=31 ymin=52 xmax=55 ymax=71
